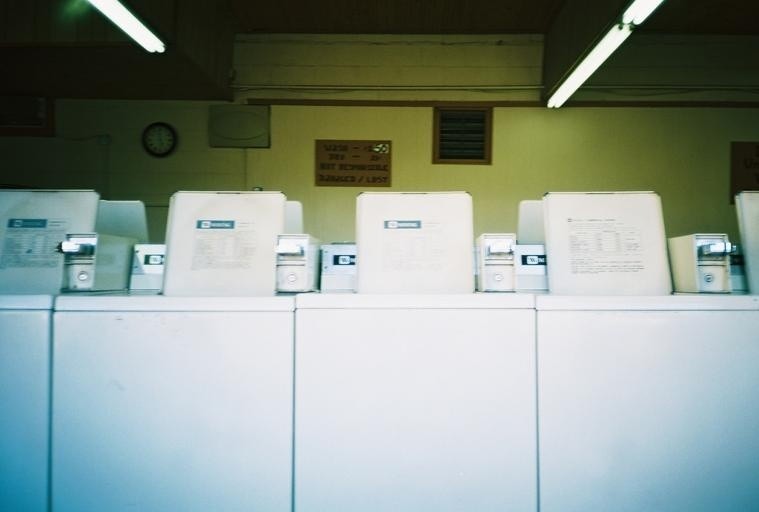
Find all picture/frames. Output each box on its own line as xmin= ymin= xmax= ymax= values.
xmin=428 ymin=102 xmax=496 ymax=166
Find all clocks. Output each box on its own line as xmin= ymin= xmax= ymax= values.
xmin=141 ymin=120 xmax=176 ymax=159
